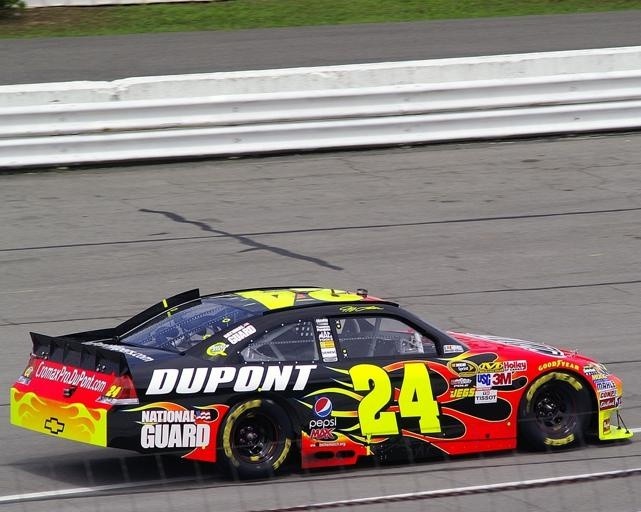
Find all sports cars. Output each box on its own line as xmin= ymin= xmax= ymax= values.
xmin=8 ymin=287 xmax=634 ymax=481
